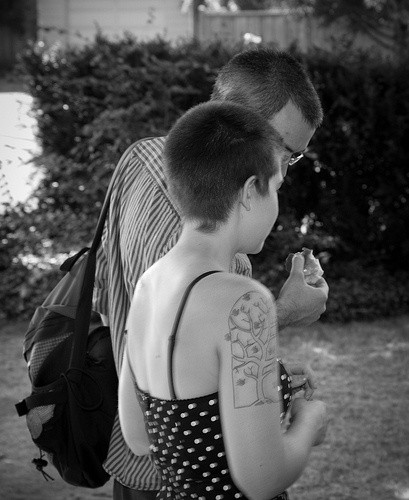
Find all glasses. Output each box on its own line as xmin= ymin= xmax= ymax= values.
xmin=284 ymin=152 xmax=302 ymax=165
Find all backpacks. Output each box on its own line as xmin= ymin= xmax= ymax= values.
xmin=15 ymin=137 xmax=115 ymax=487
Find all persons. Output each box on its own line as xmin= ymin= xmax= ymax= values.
xmin=91 ymin=44 xmax=331 ymax=499
xmin=115 ymin=97 xmax=329 ymax=499
